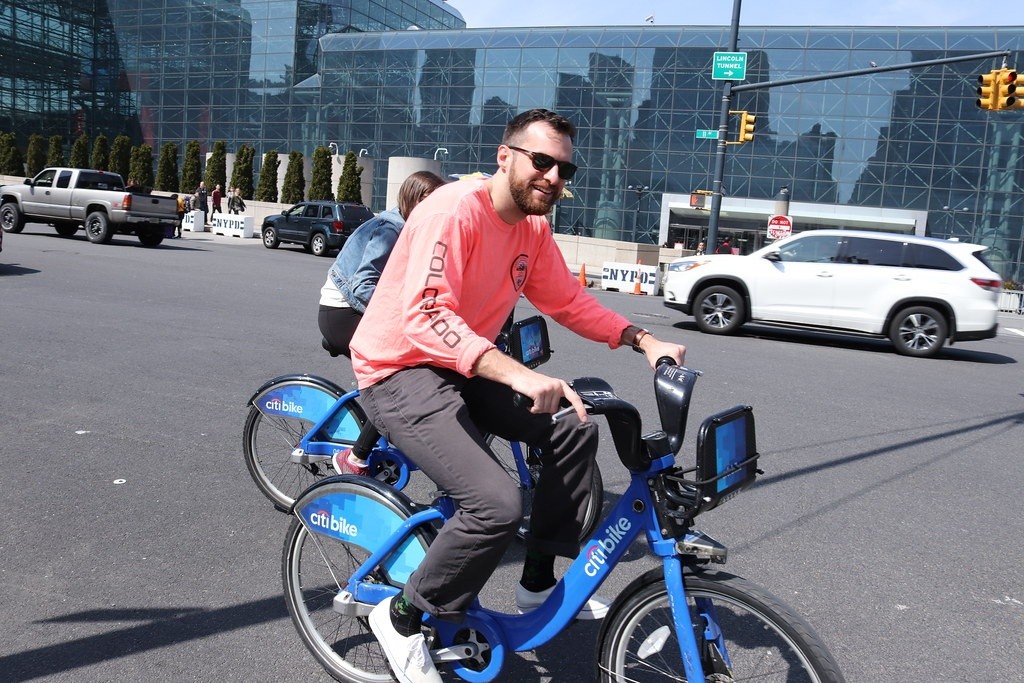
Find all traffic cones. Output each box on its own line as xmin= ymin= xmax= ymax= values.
xmin=629 ymin=268 xmax=646 ymax=295
xmin=577 ymin=263 xmax=587 ymax=288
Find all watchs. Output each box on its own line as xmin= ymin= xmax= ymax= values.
xmin=632 ymin=328 xmax=655 ymax=354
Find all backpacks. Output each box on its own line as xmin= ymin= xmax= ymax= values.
xmin=180 ymin=196 xmax=191 ymax=214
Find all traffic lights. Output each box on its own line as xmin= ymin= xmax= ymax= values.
xmin=976 ymin=71 xmax=996 ymax=111
xmin=739 ymin=113 xmax=756 ymax=142
xmin=1014 ymin=73 xmax=1024 ymax=109
xmin=998 ymin=71 xmax=1017 ymax=110
xmin=690 ymin=193 xmax=705 ymax=207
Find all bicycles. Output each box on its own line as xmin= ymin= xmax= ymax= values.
xmin=242 ymin=317 xmax=603 ymax=546
xmin=281 ymin=356 xmax=844 ymax=683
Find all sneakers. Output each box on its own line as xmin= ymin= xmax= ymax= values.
xmin=331 ymin=448 xmax=369 ymax=478
xmin=514 ymin=580 xmax=612 ymax=620
xmin=368 ymin=595 xmax=443 ymax=683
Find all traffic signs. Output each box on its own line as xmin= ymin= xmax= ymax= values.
xmin=712 ymin=51 xmax=747 ymax=80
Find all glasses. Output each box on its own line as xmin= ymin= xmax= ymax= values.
xmin=507 ymin=146 xmax=578 ymax=181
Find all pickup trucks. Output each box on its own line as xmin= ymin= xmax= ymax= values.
xmin=0 ymin=167 xmax=180 ymax=246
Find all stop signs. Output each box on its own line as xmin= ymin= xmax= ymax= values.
xmin=767 ymin=215 xmax=793 ymax=239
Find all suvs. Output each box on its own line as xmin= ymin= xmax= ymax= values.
xmin=262 ymin=202 xmax=375 ymax=256
xmin=661 ymin=227 xmax=1002 ymax=358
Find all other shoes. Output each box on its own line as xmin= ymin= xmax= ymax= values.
xmin=175 ymin=236 xmax=181 ymax=239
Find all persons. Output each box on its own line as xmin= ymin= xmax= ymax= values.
xmin=195 ymin=181 xmax=209 ymax=225
xmin=349 ymin=110 xmax=688 ymax=683
xmin=171 ymin=194 xmax=184 ymax=238
xmin=694 ymin=242 xmax=706 ymax=255
xmin=317 ymin=171 xmax=446 ymax=476
xmin=228 ymin=187 xmax=247 ymax=214
xmin=210 ymin=184 xmax=222 ymax=221
xmin=716 ymin=237 xmax=734 ymax=254
